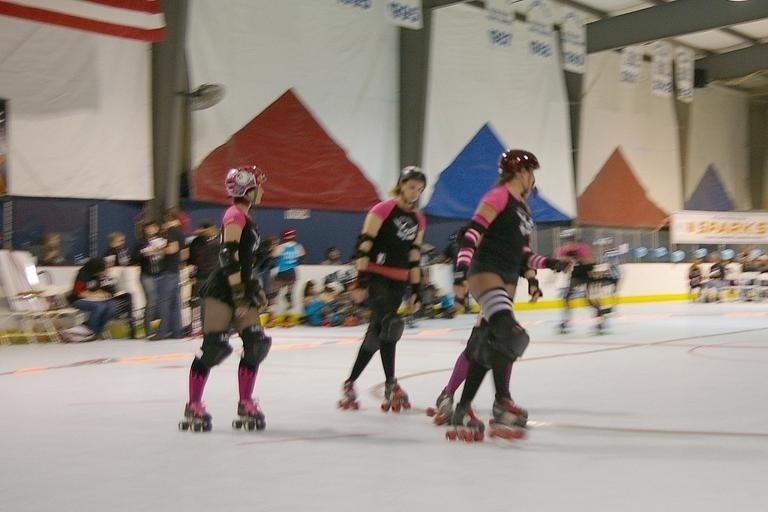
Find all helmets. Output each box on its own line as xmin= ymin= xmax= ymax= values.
xmin=559 ymin=230 xmax=574 ymax=242
xmin=226 ymin=166 xmax=267 ymax=198
xmin=399 ymin=166 xmax=427 ymax=182
xmin=499 ymin=150 xmax=539 ymax=177
xmin=281 ymin=228 xmax=296 ymax=241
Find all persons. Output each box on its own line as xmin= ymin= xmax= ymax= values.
xmin=337 ymin=164 xmax=427 ymax=403
xmin=254 ymin=227 xmax=370 ymax=328
xmin=403 ymin=231 xmax=480 ymax=329
xmin=551 ymin=225 xmax=622 ymax=339
xmin=32 ymin=207 xmax=219 ymax=342
xmin=182 ymin=162 xmax=274 ymax=423
xmin=432 ymin=177 xmax=543 ymax=428
xmin=688 ymin=246 xmax=768 ymax=303
xmin=450 ymin=147 xmax=574 ymax=431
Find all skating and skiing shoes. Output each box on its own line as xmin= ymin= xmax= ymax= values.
xmin=427 ymin=388 xmax=454 ymax=425
xmin=178 ymin=401 xmax=212 ymax=431
xmin=447 ymin=403 xmax=485 ymax=441
xmin=490 ymin=393 xmax=529 ymax=439
xmin=381 ymin=377 xmax=411 ymax=410
xmin=231 ymin=399 xmax=266 ymax=429
xmin=339 ymin=380 xmax=358 ymax=410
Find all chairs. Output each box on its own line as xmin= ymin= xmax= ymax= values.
xmin=2 ymin=249 xmax=78 ymax=347
